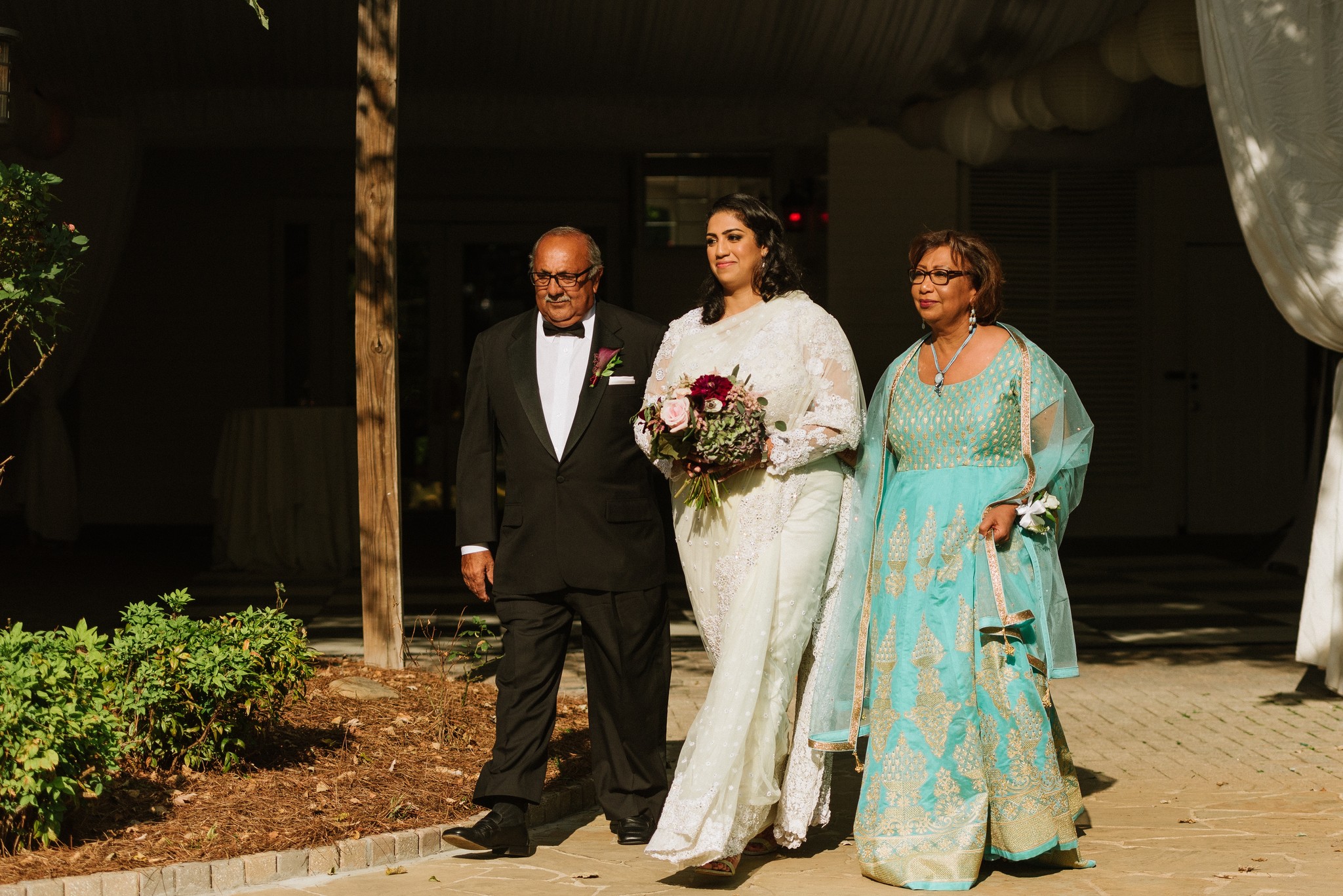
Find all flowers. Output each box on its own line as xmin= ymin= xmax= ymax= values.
xmin=589 ymin=347 xmax=624 ymax=387
xmin=629 ymin=364 xmax=787 ymax=512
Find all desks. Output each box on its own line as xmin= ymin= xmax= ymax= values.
xmin=210 ymin=405 xmax=357 ymax=575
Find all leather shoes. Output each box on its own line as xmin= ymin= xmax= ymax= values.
xmin=617 ymin=817 xmax=652 ymax=844
xmin=441 ymin=811 xmax=530 ymax=857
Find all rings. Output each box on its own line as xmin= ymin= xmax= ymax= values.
xmin=995 ymin=543 xmax=1002 ymax=549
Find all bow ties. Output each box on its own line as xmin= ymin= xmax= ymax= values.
xmin=543 ymin=321 xmax=585 ymax=339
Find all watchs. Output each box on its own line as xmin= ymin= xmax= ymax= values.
xmin=760 ymin=438 xmax=769 ymax=468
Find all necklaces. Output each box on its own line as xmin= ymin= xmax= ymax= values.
xmin=930 ymin=323 xmax=977 ymax=397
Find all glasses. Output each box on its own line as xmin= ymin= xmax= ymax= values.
xmin=531 ymin=264 xmax=597 ymax=287
xmin=908 ymin=268 xmax=977 ymax=285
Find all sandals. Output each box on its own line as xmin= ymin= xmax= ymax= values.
xmin=696 ymin=857 xmax=736 ymax=877
xmin=743 ymin=836 xmax=778 ymax=857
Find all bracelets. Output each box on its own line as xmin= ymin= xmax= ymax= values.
xmin=1014 ymin=491 xmax=1061 ymax=535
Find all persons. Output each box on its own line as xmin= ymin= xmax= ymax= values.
xmin=809 ymin=229 xmax=1094 ymax=890
xmin=441 ymin=227 xmax=670 ymax=858
xmin=631 ymin=192 xmax=869 ymax=877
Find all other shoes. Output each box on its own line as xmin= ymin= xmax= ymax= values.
xmin=1295 ymin=680 xmax=1343 ymax=700
